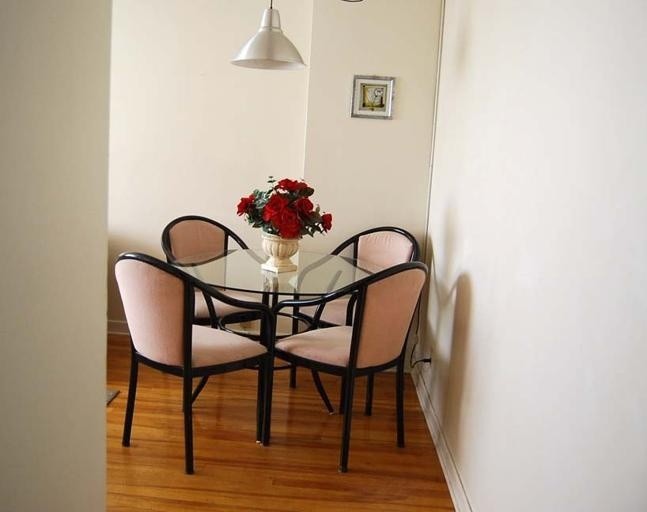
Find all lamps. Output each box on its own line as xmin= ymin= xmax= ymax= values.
xmin=227 ymin=1 xmax=306 ymax=71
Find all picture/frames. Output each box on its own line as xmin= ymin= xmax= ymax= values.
xmin=349 ymin=74 xmax=394 ymax=120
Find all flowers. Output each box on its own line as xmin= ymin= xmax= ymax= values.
xmin=234 ymin=174 xmax=332 ymax=240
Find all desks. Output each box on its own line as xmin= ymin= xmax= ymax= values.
xmin=166 ymin=248 xmax=387 ymax=449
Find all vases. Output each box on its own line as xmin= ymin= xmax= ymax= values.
xmin=258 ymin=231 xmax=298 ymax=274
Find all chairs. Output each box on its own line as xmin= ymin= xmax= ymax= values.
xmin=112 ymin=251 xmax=274 ymax=475
xmin=260 ymin=262 xmax=430 ymax=473
xmin=290 ymin=226 xmax=419 ymax=416
xmin=161 ymin=214 xmax=269 ymax=330
